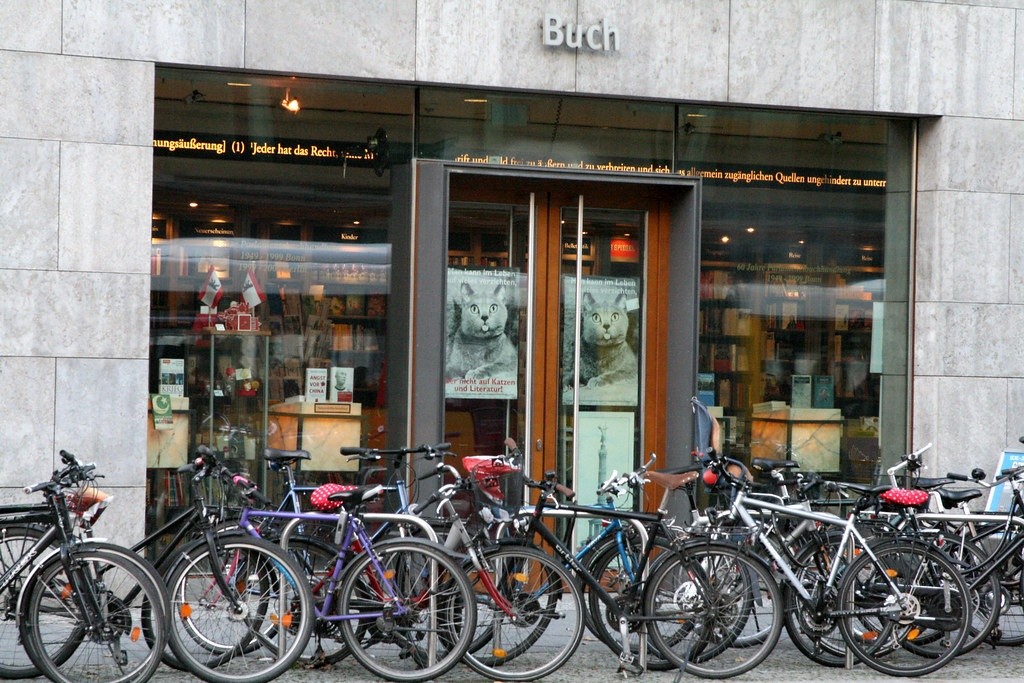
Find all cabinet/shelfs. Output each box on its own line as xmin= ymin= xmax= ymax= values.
xmin=700 ymin=260 xmax=881 ymax=420
xmin=448 ymin=231 xmax=595 ymax=280
xmin=152 ymin=212 xmax=391 ymax=408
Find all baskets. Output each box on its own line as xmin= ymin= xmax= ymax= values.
xmin=467 ymin=470 xmax=521 ymax=526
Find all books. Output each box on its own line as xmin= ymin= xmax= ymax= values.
xmin=277 ymin=280 xmax=385 ymax=376
xmin=329 ymin=367 xmax=354 ymax=403
xmin=700 ymin=266 xmax=884 ymax=447
xmin=158 ymin=357 xmax=185 ymax=396
xmin=305 ymin=368 xmax=327 ymax=402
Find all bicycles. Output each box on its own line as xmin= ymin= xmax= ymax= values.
xmin=0 ymin=436 xmax=1024 ymax=677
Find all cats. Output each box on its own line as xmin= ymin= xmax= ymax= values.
xmin=445 ymin=282 xmax=518 ymax=381
xmin=564 ymin=291 xmax=638 ymax=389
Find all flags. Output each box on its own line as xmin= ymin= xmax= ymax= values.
xmin=240 ymin=269 xmax=265 ymax=309
xmin=197 ymin=266 xmax=224 ymax=308
